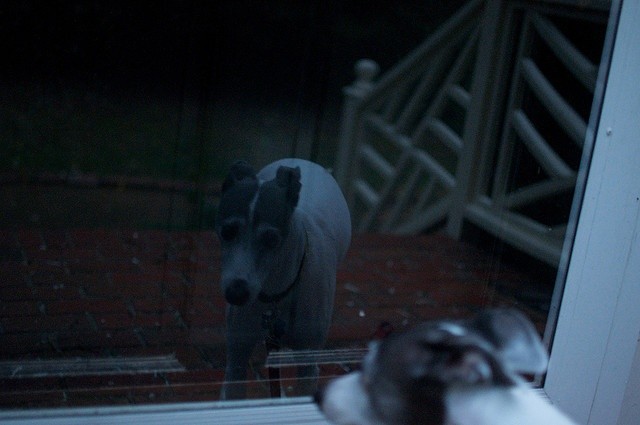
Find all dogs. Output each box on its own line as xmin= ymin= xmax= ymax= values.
xmin=217 ymin=156 xmax=353 ymax=400
xmin=312 ymin=304 xmax=577 ymax=425
